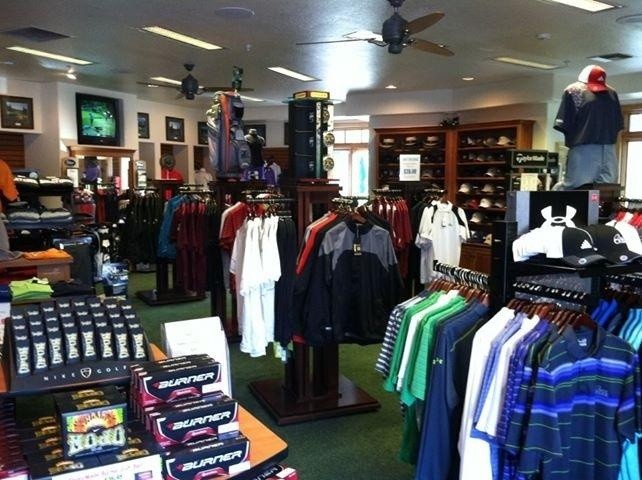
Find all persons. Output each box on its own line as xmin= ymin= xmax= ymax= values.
xmin=81 ymin=99 xmax=115 ymax=137
xmin=244 ymin=128 xmax=267 ymax=170
xmin=552 ymin=62 xmax=625 ymax=191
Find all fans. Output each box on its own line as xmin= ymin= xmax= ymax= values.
xmin=135 ymin=62 xmax=255 ymax=101
xmin=294 ymin=0 xmax=457 ymax=60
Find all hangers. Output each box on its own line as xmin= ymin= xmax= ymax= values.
xmin=421 ymin=263 xmax=641 ymax=333
xmin=126 ymin=188 xmax=367 ymax=226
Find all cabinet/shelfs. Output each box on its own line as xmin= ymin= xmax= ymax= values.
xmin=374 ymin=120 xmax=538 ymax=273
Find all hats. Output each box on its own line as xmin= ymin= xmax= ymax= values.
xmin=578 ymin=64 xmax=609 ymax=93
xmin=381 ymin=136 xmax=512 ymax=245
xmin=514 ymin=220 xmax=640 ymax=268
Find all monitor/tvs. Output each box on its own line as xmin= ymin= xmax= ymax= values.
xmin=198 ymin=121 xmax=208 ymax=144
